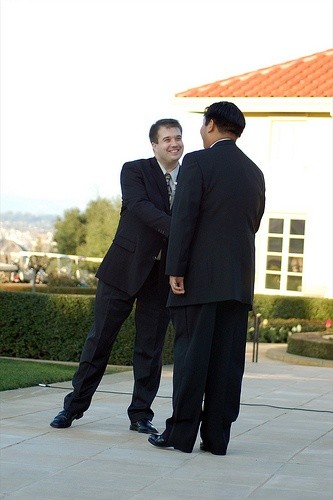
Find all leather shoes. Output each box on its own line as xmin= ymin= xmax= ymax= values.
xmin=148 ymin=433 xmax=172 ymax=446
xmin=129 ymin=419 xmax=160 ymax=435
xmin=199 ymin=442 xmax=207 ymax=450
xmin=50 ymin=409 xmax=84 ymax=429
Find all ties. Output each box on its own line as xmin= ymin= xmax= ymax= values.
xmin=165 ymin=173 xmax=172 ymax=209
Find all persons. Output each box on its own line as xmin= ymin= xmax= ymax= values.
xmin=51 ymin=119 xmax=186 ymax=434
xmin=147 ymin=101 xmax=266 ymax=455
xmin=11 ymin=264 xmax=49 ymax=284
